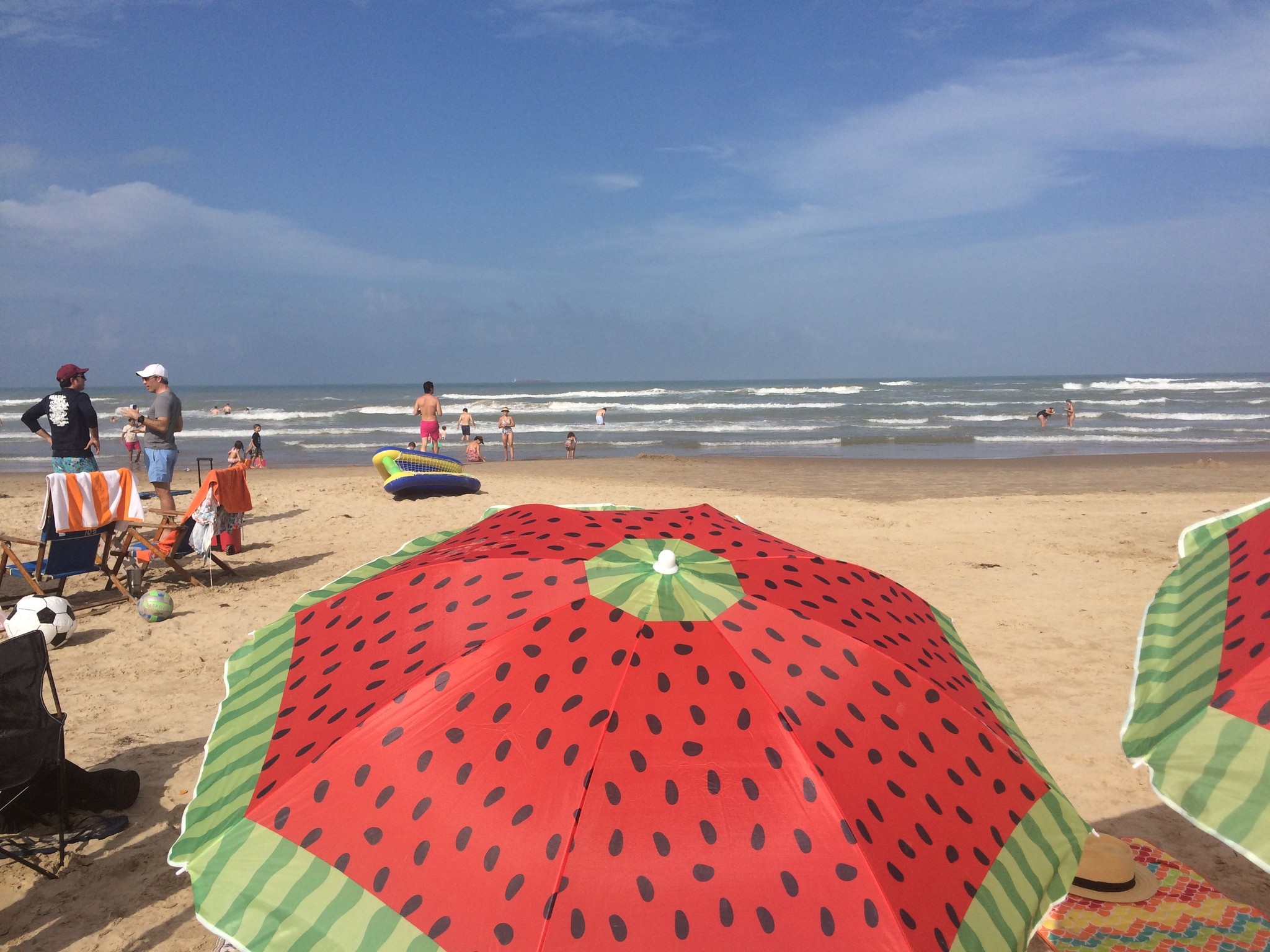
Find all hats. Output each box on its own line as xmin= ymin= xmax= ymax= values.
xmin=476 ymin=435 xmax=484 ymax=445
xmin=501 ymin=407 xmax=510 ymax=412
xmin=1069 ymin=834 xmax=1159 ymax=902
xmin=1066 ymin=399 xmax=1073 ymax=403
xmin=136 ymin=363 xmax=168 ymax=377
xmin=56 ymin=363 xmax=89 ymax=383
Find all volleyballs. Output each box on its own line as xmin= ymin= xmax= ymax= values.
xmin=137 ymin=590 xmax=174 ymax=622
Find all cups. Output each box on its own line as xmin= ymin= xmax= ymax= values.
xmin=127 ymin=569 xmax=142 ymax=597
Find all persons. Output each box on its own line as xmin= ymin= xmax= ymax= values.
xmin=245 ymin=409 xmax=250 ymax=414
xmin=121 ymin=364 xmax=183 ymax=541
xmin=110 ymin=416 xmax=121 ymax=422
xmin=228 ymin=424 xmax=265 ymax=468
xmin=0 ymin=419 xmax=3 ymax=427
xmin=223 ymin=404 xmax=232 ymax=414
xmin=1036 ymin=408 xmax=1055 ymax=427
xmin=499 ymin=408 xmax=515 ymax=461
xmin=414 ymin=381 xmax=442 ymax=454
xmin=565 ymin=432 xmax=577 ymax=459
xmin=457 ymin=408 xmax=476 ymax=440
xmin=466 ymin=436 xmax=486 ymax=462
xmin=596 ymin=408 xmax=606 ymax=425
xmin=121 ymin=417 xmax=145 ymax=462
xmin=407 ymin=426 xmax=446 ymax=451
xmin=1063 ymin=399 xmax=1075 ymax=427
xmin=23 ymin=364 xmax=101 ymax=474
xmin=210 ymin=406 xmax=223 ymax=415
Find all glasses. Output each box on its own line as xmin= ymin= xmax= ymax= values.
xmin=72 ymin=375 xmax=85 ymax=379
xmin=143 ymin=376 xmax=157 ymax=382
xmin=503 ymin=411 xmax=507 ymax=412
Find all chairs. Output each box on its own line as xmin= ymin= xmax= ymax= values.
xmin=0 ymin=629 xmax=68 ymax=880
xmin=0 ymin=463 xmax=253 ymax=618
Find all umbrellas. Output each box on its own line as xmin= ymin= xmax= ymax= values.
xmin=1122 ymin=497 xmax=1270 ymax=886
xmin=165 ymin=503 xmax=1095 ymax=952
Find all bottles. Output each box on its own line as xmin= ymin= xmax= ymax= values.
xmin=118 ymin=546 xmax=142 ymax=551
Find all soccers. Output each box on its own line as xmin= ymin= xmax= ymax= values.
xmin=3 ymin=594 xmax=78 ymax=651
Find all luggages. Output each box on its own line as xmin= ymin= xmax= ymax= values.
xmin=197 ymin=458 xmax=241 ymax=556
